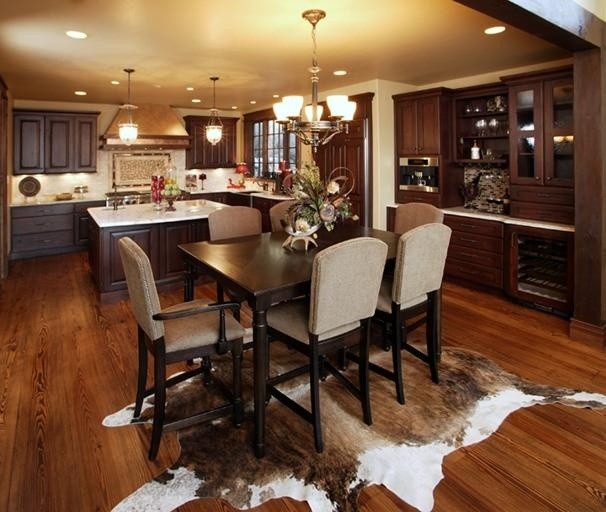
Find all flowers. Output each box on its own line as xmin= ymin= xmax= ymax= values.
xmin=280 ymin=161 xmax=361 ymax=237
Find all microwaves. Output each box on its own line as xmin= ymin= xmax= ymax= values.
xmin=399 ymin=156 xmax=438 ymax=195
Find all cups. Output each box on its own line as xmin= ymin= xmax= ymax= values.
xmin=474 ymin=119 xmax=510 ymax=137
xmin=263 ymin=184 xmax=268 ymax=191
xmin=464 ymin=104 xmax=480 ymax=113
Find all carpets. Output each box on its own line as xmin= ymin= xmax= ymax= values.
xmin=98 ymin=325 xmax=606 ymax=512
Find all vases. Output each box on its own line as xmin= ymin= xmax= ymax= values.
xmin=283 ymin=216 xmax=319 ymax=253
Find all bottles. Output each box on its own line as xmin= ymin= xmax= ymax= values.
xmin=457 ymin=140 xmax=497 ymax=161
xmin=149 ymin=164 xmax=178 ymax=210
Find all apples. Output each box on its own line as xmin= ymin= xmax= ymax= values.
xmin=161 ymin=183 xmax=181 ymax=196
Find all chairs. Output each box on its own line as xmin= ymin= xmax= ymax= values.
xmin=119 ymin=237 xmax=245 ymax=461
xmin=209 ymin=203 xmax=452 ymax=459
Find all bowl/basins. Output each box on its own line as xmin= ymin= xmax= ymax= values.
xmin=55 ymin=192 xmax=72 ymax=201
xmin=525 ymin=136 xmax=566 ymax=154
xmin=516 ymin=84 xmax=572 ymax=105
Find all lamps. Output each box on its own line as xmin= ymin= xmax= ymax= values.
xmin=197 ymin=172 xmax=206 ymax=191
xmin=270 ymin=7 xmax=356 ymax=156
xmin=205 ymin=75 xmax=223 ymax=145
xmin=225 ymin=162 xmax=249 ymax=189
xmin=116 ymin=68 xmax=139 ymax=147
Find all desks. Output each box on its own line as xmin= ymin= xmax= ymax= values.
xmin=177 ymin=226 xmax=404 ymax=460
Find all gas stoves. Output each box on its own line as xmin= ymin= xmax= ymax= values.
xmin=106 ymin=191 xmax=190 ymax=206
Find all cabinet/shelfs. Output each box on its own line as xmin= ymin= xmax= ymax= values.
xmin=182 ymin=191 xmax=229 ymax=206
xmin=8 ymin=200 xmax=75 ymax=262
xmin=182 ymin=115 xmax=240 ymax=170
xmin=451 ymin=81 xmax=506 ymax=164
xmin=75 ymin=199 xmax=106 ymax=253
xmin=160 ymin=218 xmax=211 ymax=284
xmin=391 ymin=87 xmax=456 ymax=156
xmin=12 ymin=108 xmax=73 ymax=174
xmin=509 ymin=220 xmax=574 ymax=319
xmin=229 ymin=192 xmax=273 ymax=233
xmin=306 ymin=91 xmax=375 ymax=227
xmin=87 ymin=214 xmax=160 ymax=303
xmin=444 ymin=215 xmax=508 ymax=291
xmin=73 ymin=110 xmax=102 ymax=174
xmin=500 ymin=64 xmax=576 ymax=226
xmin=396 ymin=192 xmax=464 ymax=208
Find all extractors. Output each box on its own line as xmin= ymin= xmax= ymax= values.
xmin=97 ymin=104 xmax=192 ymax=151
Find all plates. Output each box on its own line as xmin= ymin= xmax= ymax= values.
xmin=18 ymin=176 xmax=40 ymax=197
